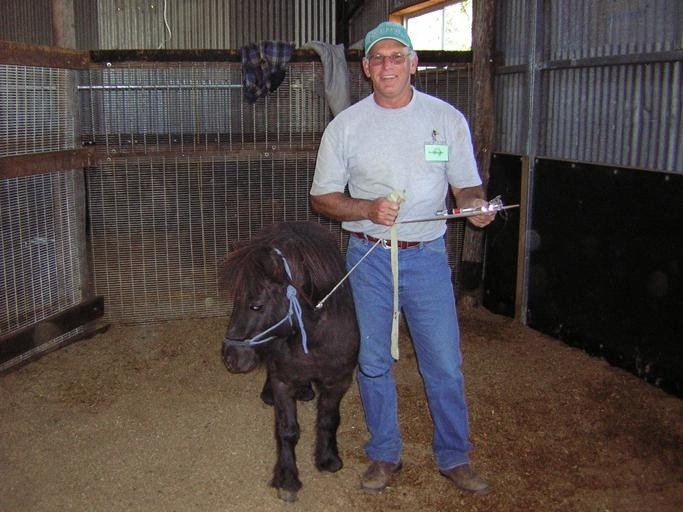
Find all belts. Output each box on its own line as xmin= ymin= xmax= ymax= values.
xmin=351 ymin=231 xmax=420 ymax=250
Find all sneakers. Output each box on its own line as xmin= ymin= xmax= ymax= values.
xmin=438 ymin=463 xmax=487 ymax=491
xmin=361 ymin=456 xmax=403 ymax=491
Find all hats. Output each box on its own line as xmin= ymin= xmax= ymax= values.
xmin=362 ymin=21 xmax=413 ymax=56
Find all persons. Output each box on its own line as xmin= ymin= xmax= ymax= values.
xmin=307 ymin=20 xmax=497 ymax=494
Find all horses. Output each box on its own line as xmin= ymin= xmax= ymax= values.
xmin=215 ymin=219 xmax=361 ymax=503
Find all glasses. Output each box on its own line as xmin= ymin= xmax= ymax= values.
xmin=368 ymin=52 xmax=410 ymax=66
